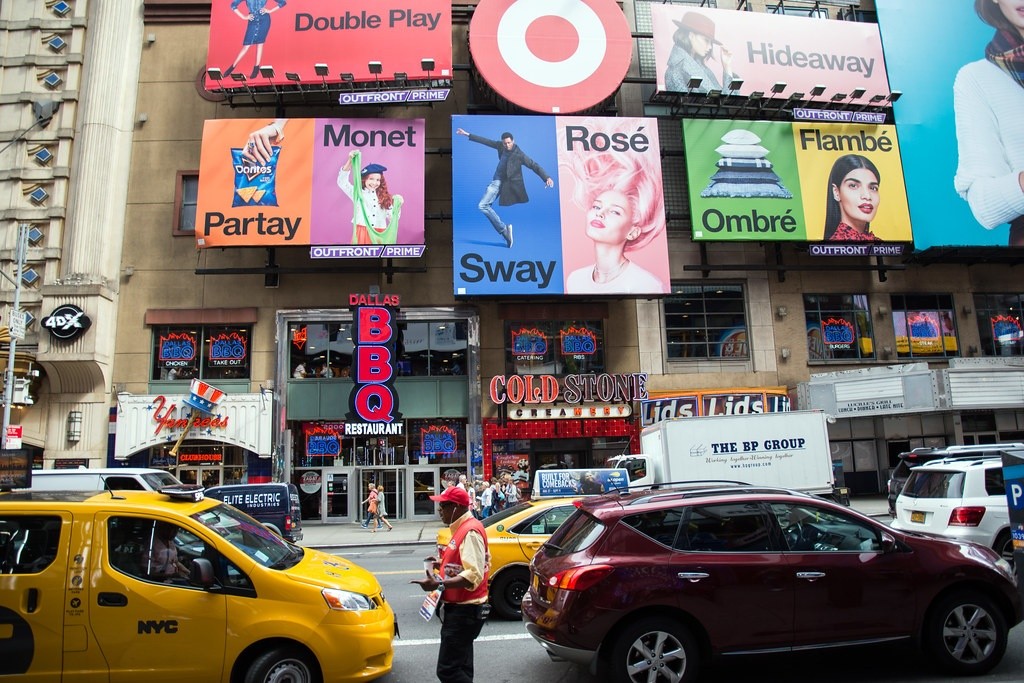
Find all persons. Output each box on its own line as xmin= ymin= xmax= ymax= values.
xmin=567 ymin=150 xmax=665 ymax=293
xmin=223 ymin=0 xmax=286 ymax=79
xmin=441 ymin=474 xmax=520 ymax=519
xmin=953 ymin=0 xmax=1024 ymax=245
xmin=456 ymin=128 xmax=553 ymax=249
xmin=167 ymin=367 xmax=182 ymax=379
xmin=574 ymin=473 xmax=605 ymax=494
xmin=242 ymin=119 xmax=288 ymax=166
xmin=665 ymin=12 xmax=739 ymax=94
xmin=337 ymin=150 xmax=404 ymax=244
xmin=293 ymin=362 xmax=333 ymax=379
xmin=823 ymin=153 xmax=882 ymax=239
xmin=143 ymin=524 xmax=191 ymax=582
xmin=409 ymin=487 xmax=490 ymax=683
xmin=361 ymin=483 xmax=383 ymax=529
xmin=370 ymin=486 xmax=393 ymax=533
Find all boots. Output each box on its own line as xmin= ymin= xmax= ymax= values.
xmin=223 ymin=65 xmax=235 ymax=77
xmin=250 ymin=65 xmax=259 ymax=79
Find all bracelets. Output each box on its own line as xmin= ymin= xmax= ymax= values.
xmin=268 ymin=122 xmax=284 ymax=145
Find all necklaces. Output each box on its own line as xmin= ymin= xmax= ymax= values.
xmin=594 ymin=259 xmax=628 ymax=274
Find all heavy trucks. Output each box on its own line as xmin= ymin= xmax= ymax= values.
xmin=604 ymin=408 xmax=834 ymax=496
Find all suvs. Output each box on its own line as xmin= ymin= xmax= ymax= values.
xmin=890 ymin=455 xmax=1017 ymax=585
xmin=0 ymin=485 xmax=401 ymax=682
xmin=886 ymin=442 xmax=1024 ymax=519
xmin=521 ymin=478 xmax=1024 ymax=683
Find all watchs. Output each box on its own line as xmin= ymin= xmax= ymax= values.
xmin=438 ymin=581 xmax=445 ymax=591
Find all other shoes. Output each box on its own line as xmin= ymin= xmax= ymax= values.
xmin=362 ymin=524 xmax=367 ymax=528
xmin=371 ymin=530 xmax=377 ymax=532
xmin=377 ymin=525 xmax=383 ymax=529
xmin=505 ymin=224 xmax=513 ymax=248
xmin=388 ymin=525 xmax=392 ymax=531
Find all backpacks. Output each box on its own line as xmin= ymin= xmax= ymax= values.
xmin=516 ymin=487 xmax=522 ymax=499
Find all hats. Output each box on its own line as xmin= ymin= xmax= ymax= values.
xmin=360 ymin=162 xmax=387 ymax=175
xmin=429 ymin=487 xmax=470 ymax=507
xmin=672 ymin=11 xmax=723 ymax=45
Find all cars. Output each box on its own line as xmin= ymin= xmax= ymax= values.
xmin=436 ymin=468 xmax=632 ymax=621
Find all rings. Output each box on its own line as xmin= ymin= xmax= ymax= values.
xmin=248 ymin=142 xmax=255 ymax=148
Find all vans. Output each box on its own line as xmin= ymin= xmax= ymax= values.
xmin=203 ymin=482 xmax=303 ymax=543
xmin=30 ymin=467 xmax=185 ymax=492
